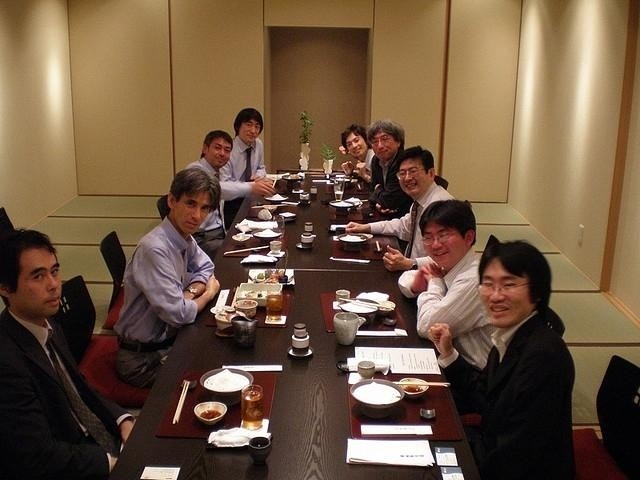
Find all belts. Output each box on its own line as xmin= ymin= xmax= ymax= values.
xmin=113 ymin=337 xmax=181 ymax=357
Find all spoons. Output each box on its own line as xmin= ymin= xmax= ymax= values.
xmin=374 ymin=241 xmax=383 ymax=252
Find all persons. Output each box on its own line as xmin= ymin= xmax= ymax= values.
xmin=219 ymin=108 xmax=265 ymax=233
xmin=345 ymin=145 xmax=456 ymax=272
xmin=0 ymin=228 xmax=135 ymax=480
xmin=398 ymin=199 xmax=496 ymax=370
xmin=367 ymin=120 xmax=412 ymax=216
xmin=341 ymin=124 xmax=375 ymax=183
xmin=175 ymin=130 xmax=277 ymax=261
xmin=427 ymin=240 xmax=577 ymax=480
xmin=113 ymin=169 xmax=221 ymax=389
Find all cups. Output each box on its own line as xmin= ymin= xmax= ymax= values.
xmin=333 ymin=175 xmax=346 ymax=202
xmin=331 ymin=310 xmax=361 ymax=345
xmin=357 ymin=361 xmax=377 ymax=378
xmin=247 ymin=436 xmax=272 ymax=464
xmin=270 ymin=240 xmax=282 ymax=253
xmin=335 ymin=289 xmax=351 ymax=303
xmin=272 ymin=214 xmax=287 ymax=238
xmin=239 ymin=384 xmax=265 ymax=433
xmin=231 ymin=316 xmax=258 ymax=348
xmin=265 ymin=285 xmax=284 ymax=321
xmin=233 ymin=299 xmax=258 ymax=318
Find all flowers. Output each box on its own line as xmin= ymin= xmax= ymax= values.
xmin=321 ymin=141 xmax=336 ymax=159
xmin=298 ymin=110 xmax=313 ymax=143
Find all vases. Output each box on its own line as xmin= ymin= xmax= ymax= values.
xmin=323 ymin=158 xmax=334 ymax=178
xmin=299 ymin=142 xmax=311 ymax=171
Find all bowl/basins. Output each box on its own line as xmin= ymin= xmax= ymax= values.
xmin=263 ymin=193 xmax=289 ymax=204
xmin=282 ymin=174 xmax=305 ymax=184
xmin=399 ymin=377 xmax=430 ymax=397
xmin=328 ymin=200 xmax=356 ymax=211
xmin=347 ymin=377 xmax=407 ymax=409
xmin=330 ymin=175 xmax=353 ymax=185
xmin=198 ymin=368 xmax=254 ymax=402
xmin=192 ymin=401 xmax=229 ymax=426
xmin=251 ymin=228 xmax=283 ymax=243
xmin=379 ymin=299 xmax=396 ymax=312
xmin=336 ymin=303 xmax=381 ymax=327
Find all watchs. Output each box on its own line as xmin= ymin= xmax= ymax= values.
xmin=184 ymin=287 xmax=201 ymax=295
xmin=410 ymin=257 xmax=418 ymax=270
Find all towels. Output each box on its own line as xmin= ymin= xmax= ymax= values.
xmin=206 ymin=427 xmax=273 ymax=449
xmin=239 ymin=254 xmax=279 ymax=264
xmin=345 ymin=196 xmax=363 ymax=209
xmin=347 ymin=356 xmax=392 ymax=385
xmin=352 ymin=291 xmax=389 ymax=309
xmin=284 ymin=172 xmax=305 ymax=180
xmin=233 ymin=219 xmax=280 ymax=233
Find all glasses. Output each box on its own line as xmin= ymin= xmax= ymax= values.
xmin=395 ymin=167 xmax=432 ymax=182
xmin=473 ymin=277 xmax=530 ymax=298
xmin=415 ymin=230 xmax=455 ymax=247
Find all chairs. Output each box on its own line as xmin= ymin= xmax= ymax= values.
xmin=548 ymin=304 xmax=566 ymax=339
xmin=98 ymin=228 xmax=126 ymax=326
xmin=156 ymin=194 xmax=170 ymax=220
xmin=573 ymin=354 xmax=639 ymax=480
xmin=50 ymin=276 xmax=150 ymax=410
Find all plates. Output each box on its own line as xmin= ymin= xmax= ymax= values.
xmin=338 ymin=231 xmax=374 ymax=246
xmin=247 ymin=268 xmax=296 ymax=285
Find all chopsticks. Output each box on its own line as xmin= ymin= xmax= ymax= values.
xmin=222 ymin=245 xmax=269 ymax=256
xmin=172 ymin=380 xmax=190 ymax=425
xmin=353 ymin=328 xmax=408 ymax=337
xmin=329 ymin=256 xmax=371 ymax=265
xmin=392 ymin=381 xmax=450 ymax=387
xmin=215 ymin=289 xmax=230 ymax=311
xmin=251 ymin=203 xmax=289 ymax=209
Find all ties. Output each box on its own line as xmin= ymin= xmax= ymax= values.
xmin=239 ymin=145 xmax=254 ymax=186
xmin=39 ymin=329 xmax=121 ymax=457
xmin=405 ymin=200 xmax=419 ymax=260
xmin=214 ymin=168 xmax=228 ymax=223
